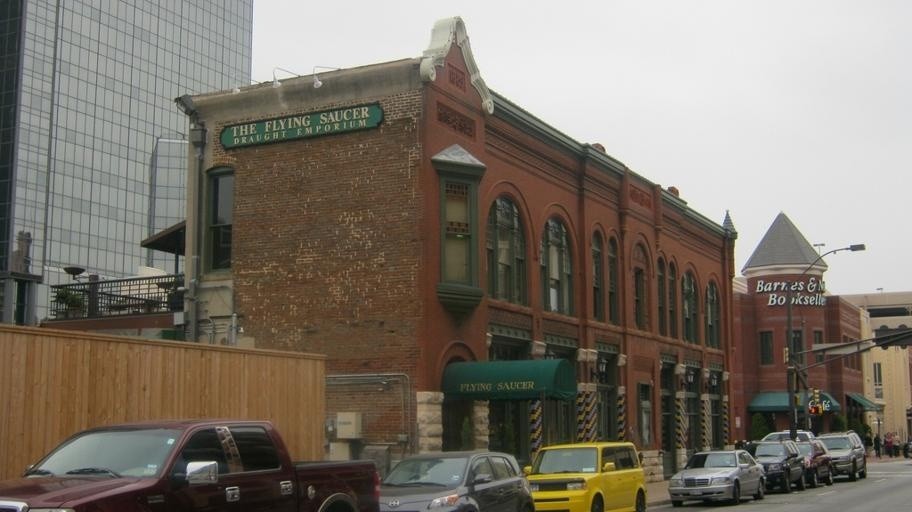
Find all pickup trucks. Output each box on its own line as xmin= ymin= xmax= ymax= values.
xmin=1 ymin=418 xmax=381 ymax=512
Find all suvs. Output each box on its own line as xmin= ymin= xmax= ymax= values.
xmin=518 ymin=441 xmax=647 ymax=512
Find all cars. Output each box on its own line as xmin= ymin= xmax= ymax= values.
xmin=380 ymin=450 xmax=535 ymax=512
xmin=667 ymin=428 xmax=868 ymax=505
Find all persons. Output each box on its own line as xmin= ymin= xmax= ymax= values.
xmin=865 ymin=429 xmax=902 ymax=459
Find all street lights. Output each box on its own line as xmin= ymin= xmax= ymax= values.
xmin=787 ymin=242 xmax=867 ymax=440
xmin=812 ymin=243 xmax=826 ymax=256
xmin=874 ymin=287 xmax=883 ymax=292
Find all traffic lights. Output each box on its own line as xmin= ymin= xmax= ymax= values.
xmin=813 ymin=389 xmax=821 ymax=403
xmin=809 ymin=405 xmax=820 ymax=415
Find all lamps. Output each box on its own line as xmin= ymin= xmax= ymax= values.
xmin=686 ymin=366 xmax=695 ymax=383
xmin=486 ymin=332 xmax=493 ymax=347
xmin=617 ymin=353 xmax=627 ymax=366
xmin=313 ymin=66 xmax=343 ymax=89
xmin=577 ymin=348 xmax=587 ymax=362
xmin=272 ymin=67 xmax=300 ymax=88
xmin=532 ymin=340 xmax=547 ymax=355
xmin=233 ymin=74 xmax=263 ymax=95
xmin=587 ymin=348 xmax=598 ymax=362
xmin=710 ymin=370 xmax=717 ymax=386
